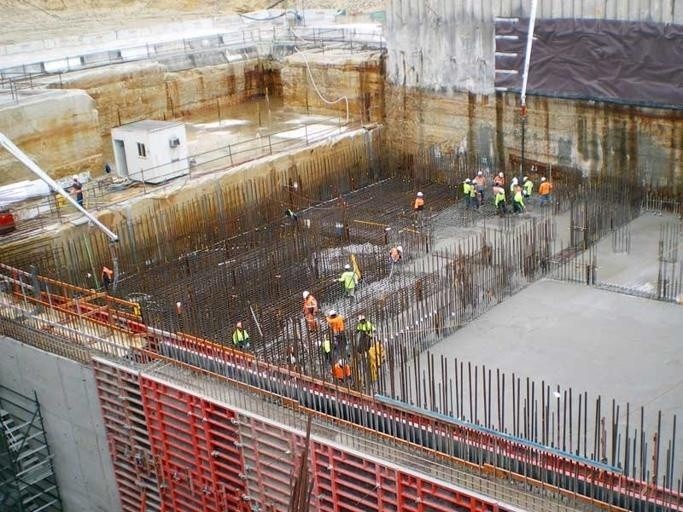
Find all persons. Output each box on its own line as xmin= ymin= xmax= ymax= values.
xmin=414 ymin=192 xmax=424 ymax=228
xmin=103 ymin=272 xmax=112 ymax=296
xmin=232 ymin=322 xmax=250 ymax=351
xmin=303 ymin=291 xmax=386 ymax=387
xmin=390 ymin=246 xmax=402 ymax=275
xmin=333 ymin=264 xmax=359 ymax=308
xmin=463 ymin=171 xmax=552 ymax=217
xmin=72 ymin=178 xmax=83 ymax=212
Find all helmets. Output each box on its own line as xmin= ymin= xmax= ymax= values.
xmin=303 ymin=290 xmax=310 ymax=299
xmin=314 ymin=310 xmax=381 ymax=368
xmin=396 ymin=245 xmax=403 ymax=253
xmin=417 ymin=192 xmax=424 ymax=196
xmin=237 ymin=322 xmax=244 ymax=328
xmin=344 ymin=263 xmax=351 ymax=268
xmin=464 ymin=170 xmax=548 ymax=198
